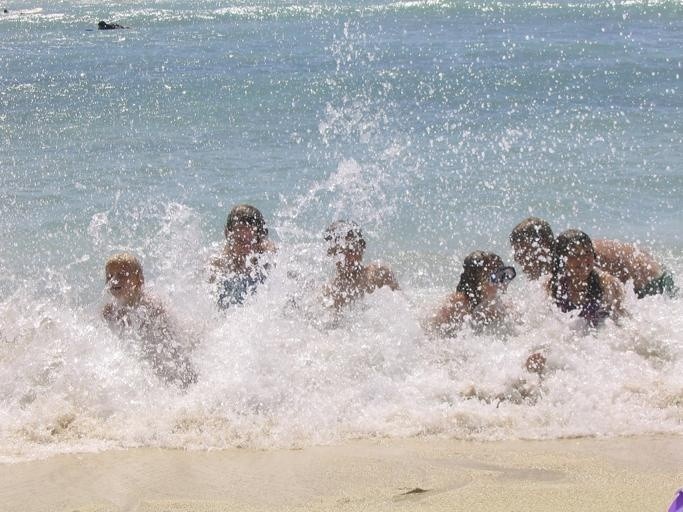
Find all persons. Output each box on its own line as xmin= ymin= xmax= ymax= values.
xmin=512 ymin=219 xmax=678 ymax=300
xmin=324 ymin=220 xmax=400 ymax=309
xmin=207 ymin=205 xmax=276 ymax=309
xmin=434 ymin=251 xmax=516 ymax=330
xmin=541 ymin=229 xmax=625 ymax=329
xmin=104 ymin=257 xmax=165 ymax=361
xmin=98 ymin=21 xmax=128 ymax=29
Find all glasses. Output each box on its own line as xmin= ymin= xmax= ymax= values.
xmin=489 ymin=265 xmax=516 ymax=283
